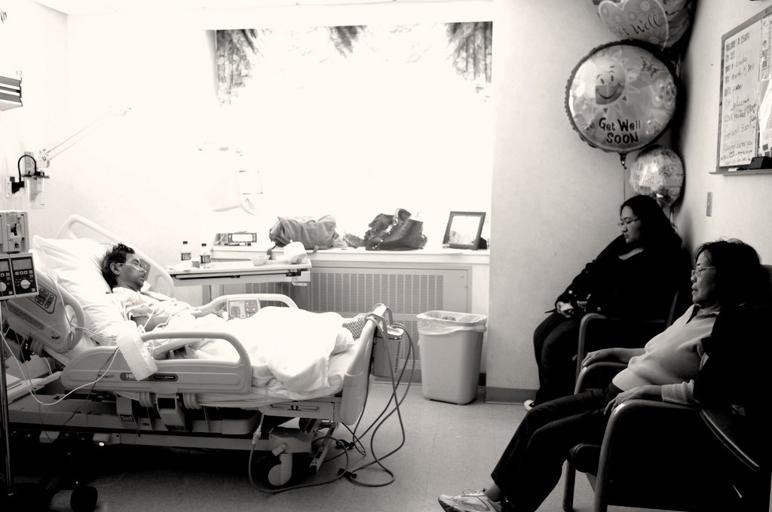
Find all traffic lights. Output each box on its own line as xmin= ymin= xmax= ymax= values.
xmin=29 ymin=235 xmax=151 ymax=346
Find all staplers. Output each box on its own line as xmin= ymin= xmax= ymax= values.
xmin=749 ymin=157 xmax=772 ymax=169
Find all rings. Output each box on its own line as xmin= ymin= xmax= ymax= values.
xmin=728 ymin=167 xmax=741 ymax=171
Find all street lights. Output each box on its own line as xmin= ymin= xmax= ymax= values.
xmin=364 ymin=209 xmax=427 ymax=249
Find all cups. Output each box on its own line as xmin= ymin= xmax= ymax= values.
xmin=692 ymin=265 xmax=716 ymax=278
xmin=618 ymin=217 xmax=638 ymax=228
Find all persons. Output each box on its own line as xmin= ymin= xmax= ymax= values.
xmin=439 ymin=237 xmax=760 ymax=511
xmin=523 ymin=194 xmax=680 ymax=411
xmin=100 ymin=242 xmax=362 ymax=382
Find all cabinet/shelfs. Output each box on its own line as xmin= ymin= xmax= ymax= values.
xmin=199 ymin=243 xmax=213 ymax=270
xmin=180 ymin=240 xmax=194 ymax=272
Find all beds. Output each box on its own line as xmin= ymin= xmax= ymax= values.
xmin=0 ymin=214 xmax=404 ymax=490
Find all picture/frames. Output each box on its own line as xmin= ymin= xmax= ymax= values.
xmin=442 ymin=210 xmax=486 ymax=250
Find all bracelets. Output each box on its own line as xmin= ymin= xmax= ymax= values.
xmin=249 ymin=253 xmax=271 ymax=266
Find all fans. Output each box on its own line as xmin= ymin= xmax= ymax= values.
xmin=715 ymin=5 xmax=772 ymax=174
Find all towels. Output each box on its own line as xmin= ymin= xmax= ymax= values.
xmin=417 ymin=310 xmax=487 ymax=404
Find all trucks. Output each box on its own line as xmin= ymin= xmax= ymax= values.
xmin=562 ymin=41 xmax=683 ymax=164
xmin=627 ymin=145 xmax=685 ymax=209
xmin=590 ymin=0 xmax=698 ymax=66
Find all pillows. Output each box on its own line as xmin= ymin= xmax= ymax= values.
xmin=29 ymin=235 xmax=151 ymax=346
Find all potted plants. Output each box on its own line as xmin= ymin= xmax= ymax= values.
xmin=442 ymin=210 xmax=486 ymax=250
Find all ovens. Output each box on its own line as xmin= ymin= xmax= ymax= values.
xmin=0 ymin=214 xmax=404 ymax=490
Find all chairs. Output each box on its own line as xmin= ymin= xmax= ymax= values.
xmin=561 ymin=267 xmax=772 ymax=512
xmin=439 ymin=488 xmax=503 ymax=512
xmin=524 ymin=399 xmax=536 ymax=412
